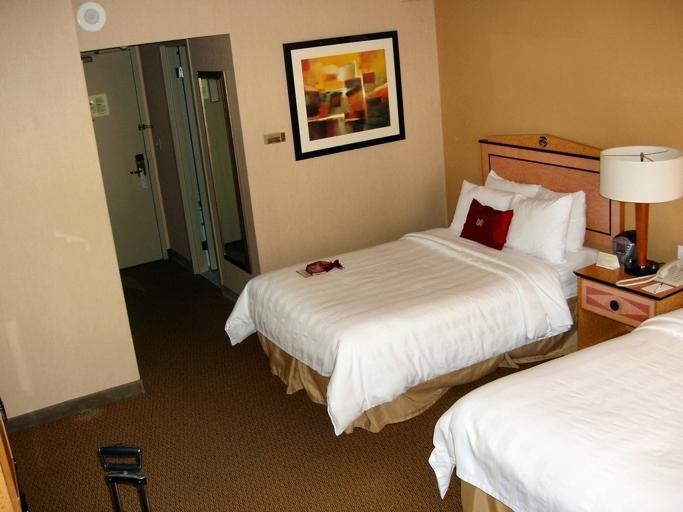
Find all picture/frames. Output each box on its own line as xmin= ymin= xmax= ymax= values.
xmin=282 ymin=30 xmax=406 ymax=161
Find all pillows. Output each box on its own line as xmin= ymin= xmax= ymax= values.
xmin=446 ymin=168 xmax=587 ymax=266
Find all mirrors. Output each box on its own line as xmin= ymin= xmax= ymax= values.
xmin=195 ymin=70 xmax=253 ymax=275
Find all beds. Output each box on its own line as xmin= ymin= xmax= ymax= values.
xmin=448 ymin=308 xmax=683 ymax=512
xmin=246 ymin=134 xmax=626 ymax=438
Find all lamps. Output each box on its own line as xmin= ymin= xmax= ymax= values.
xmin=598 ymin=144 xmax=682 ymax=277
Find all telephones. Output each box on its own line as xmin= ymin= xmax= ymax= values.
xmin=653 ymin=260 xmax=683 ymax=287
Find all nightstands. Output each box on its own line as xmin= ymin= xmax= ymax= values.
xmin=574 ymin=260 xmax=683 ymax=351
